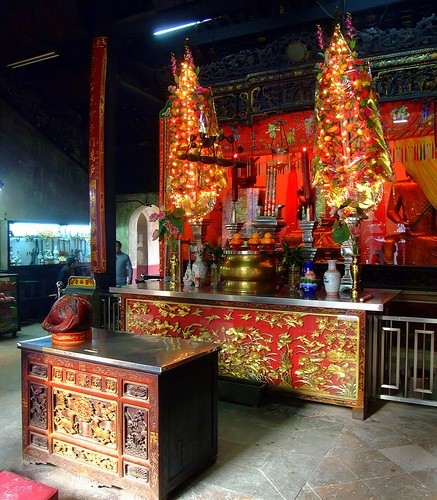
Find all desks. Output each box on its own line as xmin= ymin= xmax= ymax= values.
xmin=111 ymin=279 xmax=405 ymax=419
xmin=15 ymin=326 xmax=223 ymax=500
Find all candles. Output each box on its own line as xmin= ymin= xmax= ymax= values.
xmin=307 ymin=205 xmax=310 ymax=222
xmin=233 ymin=209 xmax=235 ymax=222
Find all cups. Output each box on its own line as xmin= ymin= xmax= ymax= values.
xmin=195 ymin=277 xmax=201 ymax=287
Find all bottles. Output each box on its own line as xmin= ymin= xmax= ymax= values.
xmin=299 ymin=261 xmax=319 ymax=295
xmin=323 ymin=260 xmax=341 ymax=295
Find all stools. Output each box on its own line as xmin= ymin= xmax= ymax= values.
xmin=0 ymin=470 xmax=58 ymax=500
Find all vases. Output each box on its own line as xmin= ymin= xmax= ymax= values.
xmin=170 ymin=252 xmax=178 ymax=289
xmin=351 ymin=255 xmax=360 ymax=299
xmin=289 ymin=267 xmax=300 ymax=289
xmin=211 ymin=264 xmax=219 ymax=288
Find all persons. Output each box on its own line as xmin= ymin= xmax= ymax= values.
xmin=382 ymin=169 xmax=437 ymax=265
xmin=116 ymin=241 xmax=133 ymax=287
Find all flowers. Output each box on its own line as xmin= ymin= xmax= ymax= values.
xmin=333 ymin=208 xmax=361 ymax=256
xmin=281 ymin=240 xmax=310 ymax=268
xmin=201 ymin=244 xmax=223 ymax=263
xmin=150 ymin=205 xmax=183 ymax=251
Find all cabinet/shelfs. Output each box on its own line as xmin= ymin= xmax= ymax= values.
xmin=0 ymin=273 xmax=22 ymax=339
